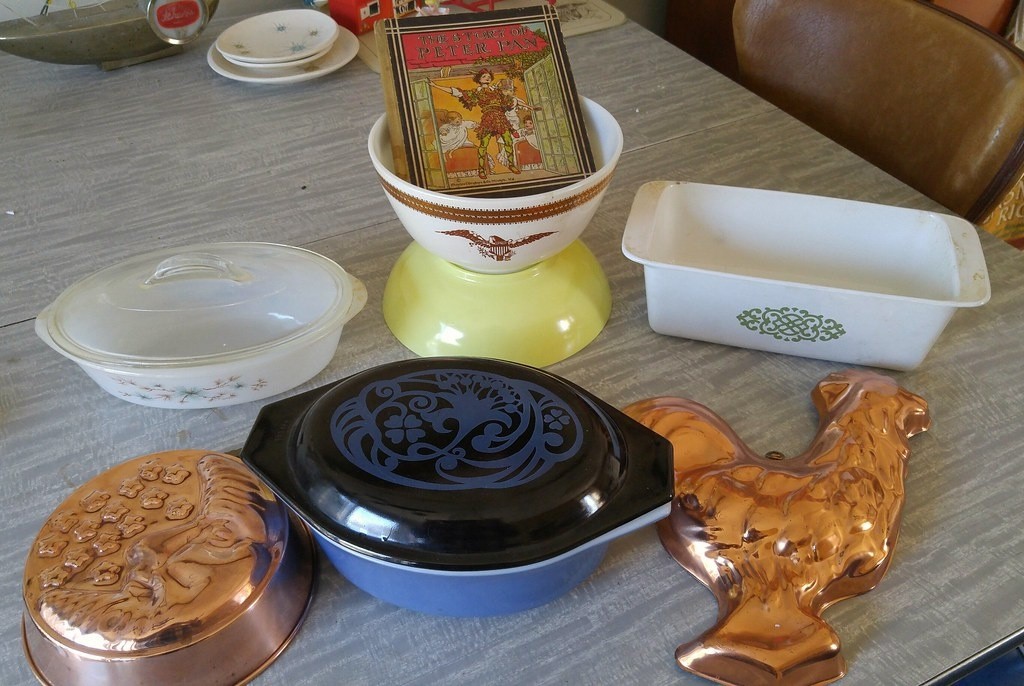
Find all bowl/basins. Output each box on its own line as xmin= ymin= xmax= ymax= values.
xmin=382 ymin=238 xmax=612 ymax=368
xmin=621 ymin=179 xmax=992 ymax=373
xmin=18 ymin=449 xmax=319 ymax=686
xmin=35 ymin=240 xmax=369 ymax=410
xmin=242 ymin=356 xmax=674 ymax=618
xmin=365 ymin=94 xmax=624 ymax=275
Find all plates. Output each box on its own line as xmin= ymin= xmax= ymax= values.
xmin=207 ymin=8 xmax=361 ymax=85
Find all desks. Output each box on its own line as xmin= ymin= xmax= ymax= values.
xmin=0 ymin=0 xmax=1024 ymax=686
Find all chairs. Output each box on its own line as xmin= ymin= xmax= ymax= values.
xmin=731 ymin=0 xmax=1024 ymax=223
